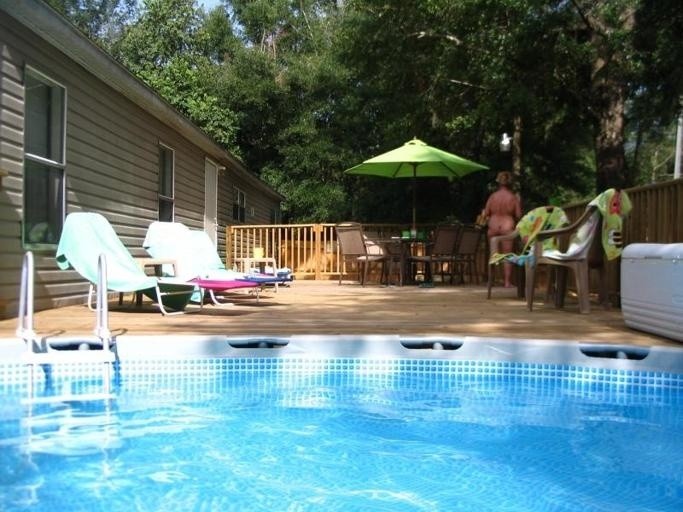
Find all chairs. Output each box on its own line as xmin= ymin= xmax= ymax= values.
xmin=57 ymin=209 xmax=290 ymax=316
xmin=484 ymin=186 xmax=627 ymax=315
xmin=333 ymin=220 xmax=484 ymax=289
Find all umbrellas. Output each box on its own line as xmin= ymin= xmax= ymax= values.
xmin=343 ymin=136 xmax=490 ymax=238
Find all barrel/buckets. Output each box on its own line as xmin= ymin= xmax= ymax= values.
xmin=253 ymin=247 xmax=264 ymax=257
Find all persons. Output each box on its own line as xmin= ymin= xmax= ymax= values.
xmin=482 ymin=172 xmax=523 ymax=288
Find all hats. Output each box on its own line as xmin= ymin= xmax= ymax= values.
xmin=496 ymin=172 xmax=512 ymax=184
